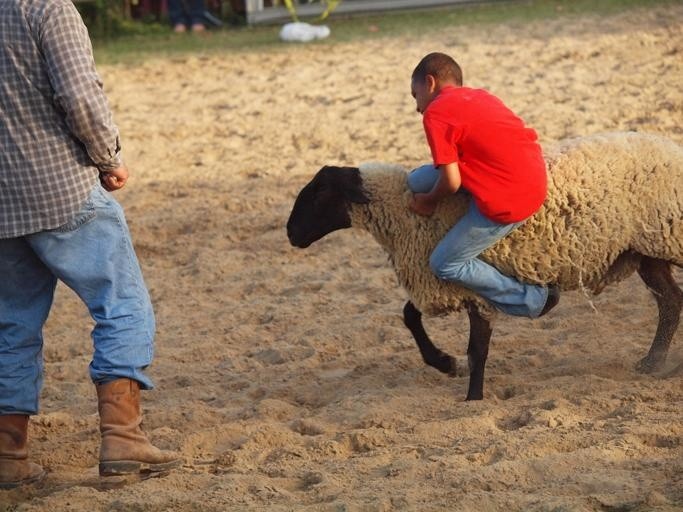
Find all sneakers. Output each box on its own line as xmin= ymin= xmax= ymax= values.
xmin=537 ymin=284 xmax=560 ymax=317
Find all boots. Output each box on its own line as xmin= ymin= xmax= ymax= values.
xmin=93 ymin=375 xmax=185 ymax=477
xmin=0 ymin=413 xmax=46 ymax=491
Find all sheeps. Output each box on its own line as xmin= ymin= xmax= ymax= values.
xmin=286 ymin=131 xmax=683 ymax=402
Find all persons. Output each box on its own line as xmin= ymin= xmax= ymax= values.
xmin=0 ymin=0 xmax=184 ymax=489
xmin=404 ymin=52 xmax=562 ymax=319
xmin=168 ymin=0 xmax=207 ymax=35
